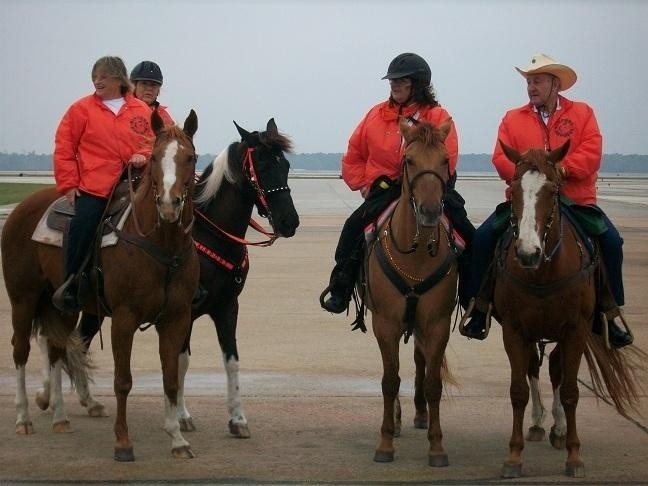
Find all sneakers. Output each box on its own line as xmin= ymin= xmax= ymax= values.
xmin=326 ymin=291 xmax=347 ymax=312
xmin=593 ymin=317 xmax=633 ymax=346
xmin=462 ymin=312 xmax=491 ymax=335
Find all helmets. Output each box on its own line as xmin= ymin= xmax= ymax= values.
xmin=131 ymin=61 xmax=162 ymax=86
xmin=382 ymin=53 xmax=430 ymax=81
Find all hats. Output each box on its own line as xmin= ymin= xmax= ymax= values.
xmin=515 ymin=53 xmax=577 ymax=91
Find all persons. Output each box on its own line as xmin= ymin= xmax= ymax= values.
xmin=458 ymin=53 xmax=634 ymax=347
xmin=51 ymin=55 xmax=160 ymax=314
xmin=329 ymin=52 xmax=477 ymax=316
xmin=127 ymin=60 xmax=176 ymax=129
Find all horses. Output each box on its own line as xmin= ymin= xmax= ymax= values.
xmin=489 ymin=133 xmax=648 ymax=482
xmin=350 ymin=112 xmax=461 ymax=471
xmin=0 ymin=106 xmax=203 ymax=463
xmin=34 ymin=116 xmax=302 ymax=442
